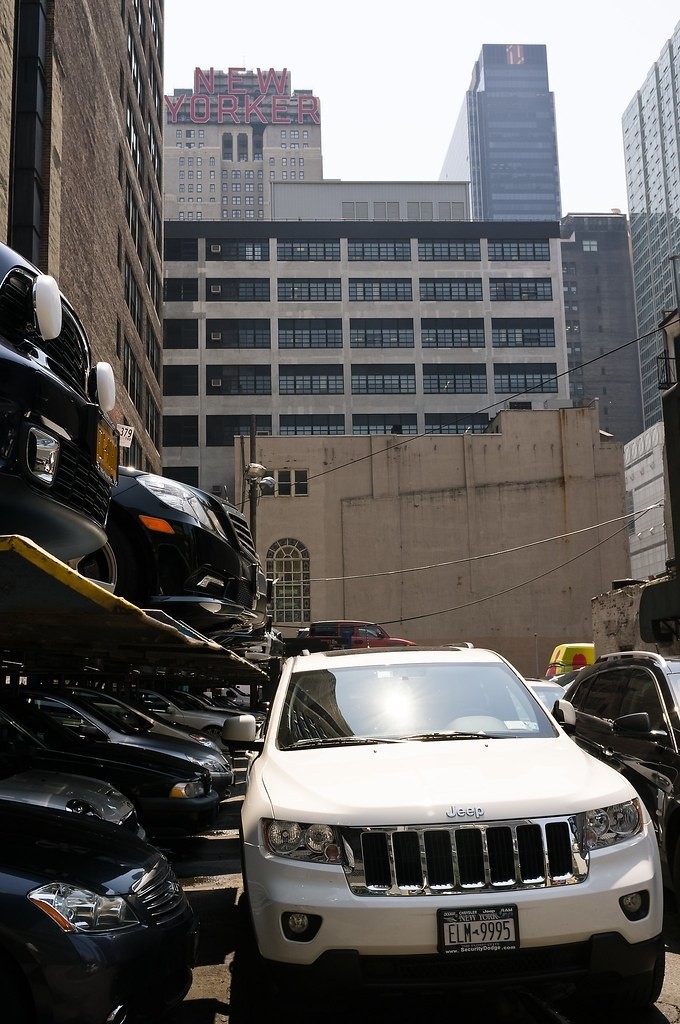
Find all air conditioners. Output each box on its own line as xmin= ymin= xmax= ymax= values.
xmin=211 ymin=285 xmax=221 ymax=294
xmin=211 ymin=332 xmax=221 ymax=340
xmin=211 ymin=245 xmax=221 ymax=252
xmin=212 ymin=379 xmax=222 ymax=387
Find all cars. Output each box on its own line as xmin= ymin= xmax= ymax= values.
xmin=507 ymin=677 xmax=570 ymax=726
xmin=0 ymin=799 xmax=199 ymax=1022
xmin=111 ymin=465 xmax=259 ymax=639
xmin=545 ymin=666 xmax=590 ymax=695
xmin=0 ymin=696 xmax=220 ymax=836
xmin=174 ymin=687 xmax=267 ymax=741
xmin=0 ymin=242 xmax=117 ymax=594
xmin=0 ymin=685 xmax=237 ymax=798
xmin=0 ymin=713 xmax=150 ymax=845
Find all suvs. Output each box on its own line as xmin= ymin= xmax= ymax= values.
xmin=551 ymin=652 xmax=680 ymax=905
xmin=309 ymin=620 xmax=416 ymax=649
xmin=220 ymin=641 xmax=665 ymax=1022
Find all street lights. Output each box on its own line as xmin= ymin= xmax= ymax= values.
xmin=241 ymin=412 xmax=276 ymax=555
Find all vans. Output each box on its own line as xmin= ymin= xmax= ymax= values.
xmin=543 ymin=643 xmax=595 ymax=684
